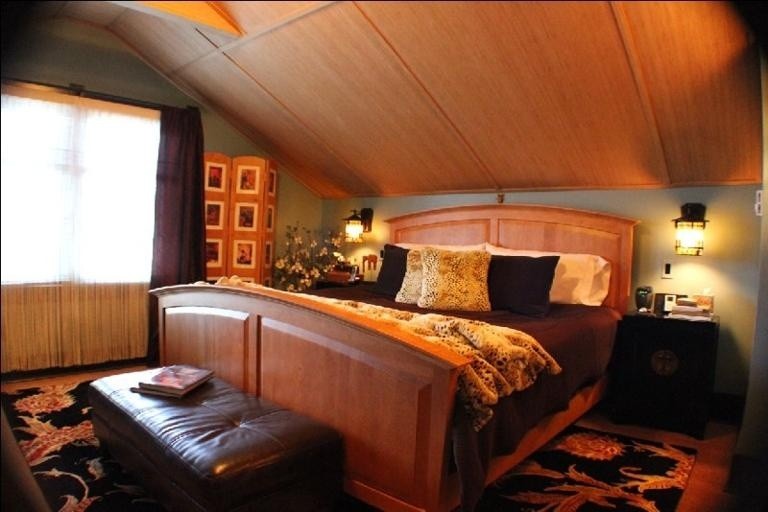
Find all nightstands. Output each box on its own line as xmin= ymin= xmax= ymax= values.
xmin=606 ymin=306 xmax=721 ymax=441
xmin=316 ymin=280 xmax=375 ymax=289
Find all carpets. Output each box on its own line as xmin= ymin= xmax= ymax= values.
xmin=0 ymin=380 xmax=699 ymax=512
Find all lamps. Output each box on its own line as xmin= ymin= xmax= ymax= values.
xmin=671 ymin=202 xmax=710 ymax=258
xmin=342 ymin=206 xmax=374 ymax=244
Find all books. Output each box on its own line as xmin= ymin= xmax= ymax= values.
xmin=130 ymin=364 xmax=215 ymax=399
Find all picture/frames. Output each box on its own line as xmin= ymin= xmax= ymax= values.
xmin=204 ymin=160 xmax=277 ymax=288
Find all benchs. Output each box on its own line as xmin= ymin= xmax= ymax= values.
xmin=87 ymin=363 xmax=346 ymax=512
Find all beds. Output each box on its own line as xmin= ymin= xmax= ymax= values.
xmin=148 ymin=205 xmax=641 ymax=512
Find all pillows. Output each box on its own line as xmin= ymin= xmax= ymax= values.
xmin=373 ymin=240 xmax=611 ymax=316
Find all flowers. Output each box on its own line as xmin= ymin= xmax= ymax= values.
xmin=273 ymin=222 xmax=347 ymax=294
